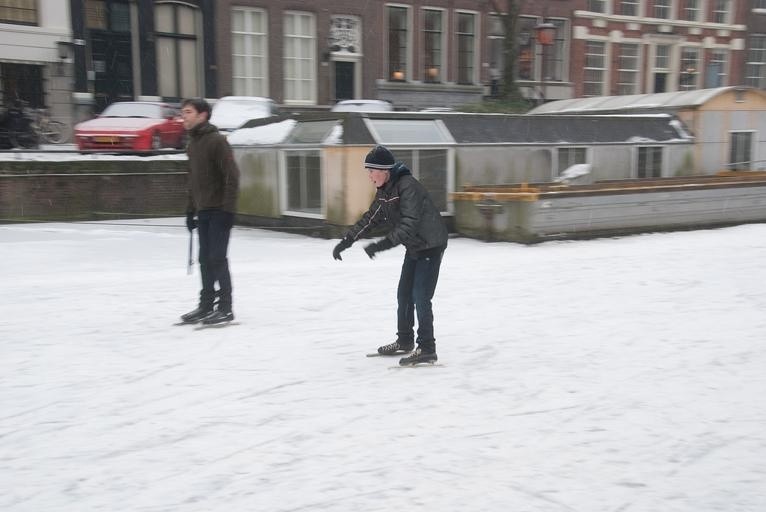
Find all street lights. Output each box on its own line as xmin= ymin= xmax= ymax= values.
xmin=532 ymin=22 xmax=557 ymax=105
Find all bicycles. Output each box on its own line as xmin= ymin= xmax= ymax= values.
xmin=27 ymin=105 xmax=70 ymax=145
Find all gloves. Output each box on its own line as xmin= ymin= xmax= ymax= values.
xmin=332 ymin=235 xmax=354 ymax=261
xmin=223 ymin=212 xmax=233 ymax=229
xmin=186 ymin=216 xmax=197 ymax=232
xmin=365 ymin=238 xmax=394 ymax=260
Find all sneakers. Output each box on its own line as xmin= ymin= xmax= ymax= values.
xmin=378 ymin=337 xmax=437 ymax=366
xmin=181 ymin=304 xmax=234 ymax=325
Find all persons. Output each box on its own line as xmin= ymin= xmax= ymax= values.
xmin=179 ymin=97 xmax=242 ymax=327
xmin=333 ymin=145 xmax=451 ymax=367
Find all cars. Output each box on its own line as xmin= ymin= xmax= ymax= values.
xmin=209 ymin=95 xmax=277 ymax=139
xmin=72 ymin=100 xmax=187 ymax=157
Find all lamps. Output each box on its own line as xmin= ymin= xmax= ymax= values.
xmin=55 ymin=40 xmax=75 ymax=73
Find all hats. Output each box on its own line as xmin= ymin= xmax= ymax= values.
xmin=364 ymin=145 xmax=396 ymax=170
